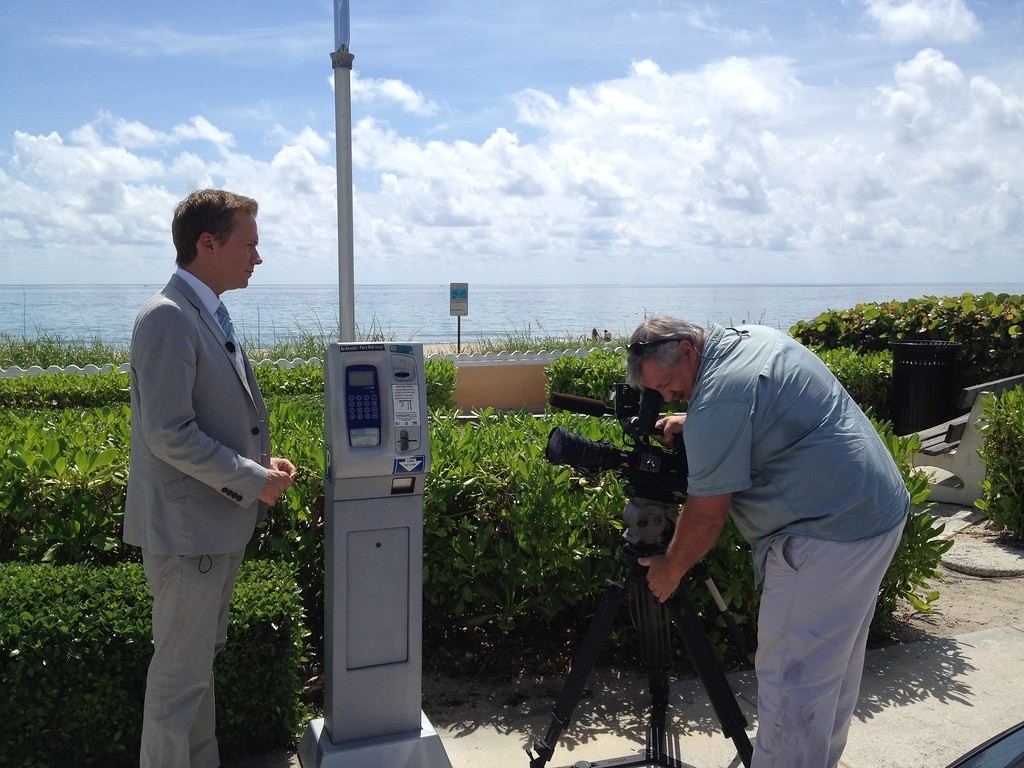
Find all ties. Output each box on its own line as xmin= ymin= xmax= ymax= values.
xmin=214 ymin=301 xmax=236 ymax=342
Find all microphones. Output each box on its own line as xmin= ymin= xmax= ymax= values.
xmin=549 ymin=391 xmax=615 ymax=417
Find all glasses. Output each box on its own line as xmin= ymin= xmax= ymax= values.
xmin=625 ymin=338 xmax=681 ymax=356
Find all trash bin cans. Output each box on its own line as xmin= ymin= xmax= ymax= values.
xmin=887 ymin=338 xmax=962 ymax=436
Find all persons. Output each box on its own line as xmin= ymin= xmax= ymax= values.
xmin=122 ymin=189 xmax=296 ymax=768
xmin=591 ymin=328 xmax=612 ymax=342
xmin=625 ymin=316 xmax=911 ymax=768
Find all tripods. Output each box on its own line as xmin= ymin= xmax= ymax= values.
xmin=529 ymin=483 xmax=755 ymax=768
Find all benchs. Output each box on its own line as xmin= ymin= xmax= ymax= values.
xmin=897 ymin=375 xmax=1023 ymax=508
